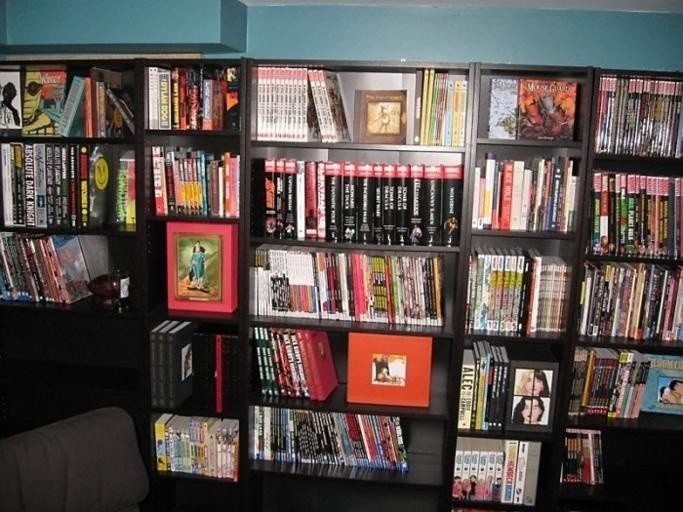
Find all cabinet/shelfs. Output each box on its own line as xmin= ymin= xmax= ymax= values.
xmin=0 ymin=54 xmax=679 ymax=510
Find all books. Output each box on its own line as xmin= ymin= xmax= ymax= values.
xmin=471 ymin=153 xmax=580 ymax=234
xmin=150 ymin=320 xmax=237 ymax=480
xmin=254 ymin=244 xmax=445 ymax=327
xmin=257 ymin=157 xmax=463 ymax=246
xmin=593 ymin=77 xmax=681 ymax=159
xmin=254 ymin=327 xmax=337 ymax=401
xmin=2 ymin=63 xmax=135 ymax=137
xmin=590 ymin=169 xmax=683 ymax=261
xmin=570 ymin=346 xmax=680 ymax=413
xmin=559 ymin=427 xmax=603 ymax=485
xmin=256 ymin=67 xmax=349 ymax=143
xmin=453 ymin=437 xmax=542 ymax=504
xmin=487 ymin=78 xmax=576 ymax=142
xmin=578 ymin=259 xmax=682 ymax=341
xmin=465 ymin=247 xmax=570 ymax=334
xmin=457 ymin=340 xmax=510 ymax=430
xmin=1 ymin=232 xmax=94 ymax=302
xmin=144 ymin=64 xmax=241 ymax=219
xmin=1 ymin=141 xmax=136 ymax=228
xmin=413 ymin=70 xmax=467 ymax=147
xmin=245 ymin=404 xmax=410 ymax=473
